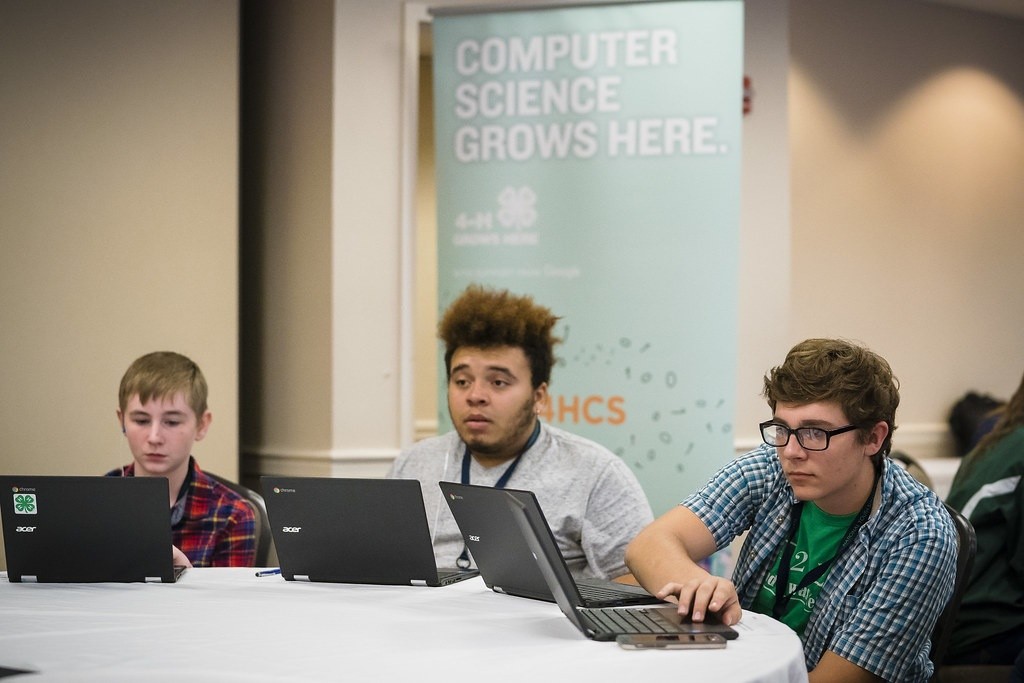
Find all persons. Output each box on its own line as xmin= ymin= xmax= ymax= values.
xmin=380 ymin=285 xmax=655 ymax=586
xmin=624 ymin=338 xmax=958 ymax=682
xmin=102 ymin=352 xmax=261 ymax=569
xmin=931 ymin=374 xmax=1024 ymax=683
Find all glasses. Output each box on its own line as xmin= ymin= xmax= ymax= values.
xmin=760 ymin=417 xmax=867 ymax=450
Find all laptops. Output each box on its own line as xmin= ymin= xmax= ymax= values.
xmin=439 ymin=480 xmax=671 ymax=608
xmin=502 ymin=490 xmax=739 ymax=643
xmin=0 ymin=475 xmax=186 ymax=584
xmin=258 ymin=475 xmax=481 ymax=587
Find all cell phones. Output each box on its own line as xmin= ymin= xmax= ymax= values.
xmin=617 ymin=634 xmax=727 ymax=651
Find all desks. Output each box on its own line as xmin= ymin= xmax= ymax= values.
xmin=0 ymin=567 xmax=809 ymax=683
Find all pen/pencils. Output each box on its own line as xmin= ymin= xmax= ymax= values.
xmin=256 ymin=569 xmax=282 ymax=578
